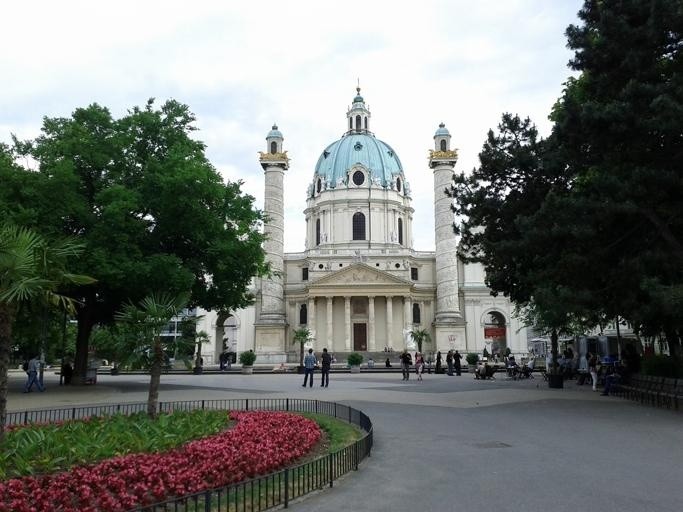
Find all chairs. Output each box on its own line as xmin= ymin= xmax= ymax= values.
xmin=610 ymin=375 xmax=683 ymax=408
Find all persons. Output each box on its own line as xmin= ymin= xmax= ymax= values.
xmin=301 ymin=348 xmax=316 ymax=388
xmin=22 ymin=352 xmax=45 ymax=393
xmin=472 ymin=349 xmax=629 ymax=397
xmin=319 ymin=347 xmax=331 ymax=387
xmin=62 ymin=351 xmax=73 ymax=385
xmin=414 ymin=349 xmax=463 ymax=384
xmin=385 ymin=357 xmax=391 ymax=367
xmin=398 ymin=348 xmax=412 ymax=380
xmin=368 ymin=358 xmax=374 ymax=368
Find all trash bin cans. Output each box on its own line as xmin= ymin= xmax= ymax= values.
xmin=85 ymin=368 xmax=97 ymax=385
